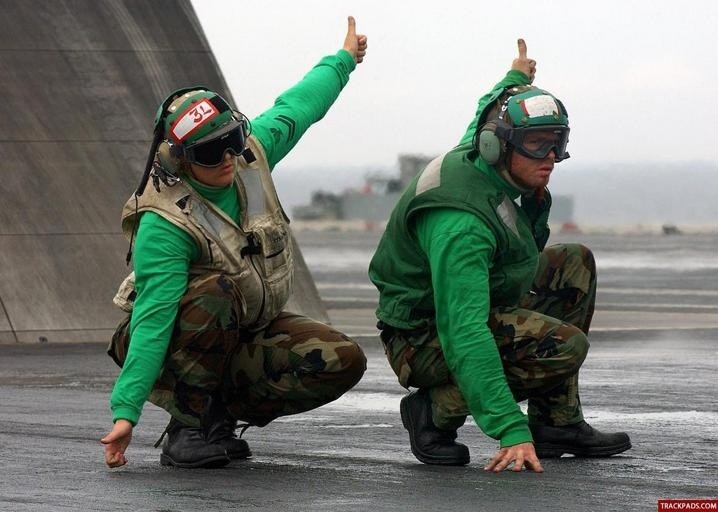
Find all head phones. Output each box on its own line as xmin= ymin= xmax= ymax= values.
xmin=478 ymin=85 xmax=517 ymax=167
xmin=154 ymin=91 xmax=248 ymax=175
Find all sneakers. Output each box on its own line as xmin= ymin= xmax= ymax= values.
xmin=159 ymin=419 xmax=230 ymax=468
xmin=528 ymin=419 xmax=632 ymax=460
xmin=199 ymin=417 xmax=253 ymax=459
xmin=400 ymin=390 xmax=470 ymax=466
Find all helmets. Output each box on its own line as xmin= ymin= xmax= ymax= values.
xmin=153 ymin=86 xmax=237 ymax=172
xmin=475 ymin=85 xmax=569 ymax=166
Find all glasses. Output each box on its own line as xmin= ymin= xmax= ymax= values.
xmin=496 ymin=122 xmax=570 ymax=162
xmin=180 ymin=119 xmax=246 ymax=168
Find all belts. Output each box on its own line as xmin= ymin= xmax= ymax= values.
xmin=379 ymin=325 xmax=402 ymax=347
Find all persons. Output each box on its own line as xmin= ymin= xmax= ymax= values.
xmin=96 ymin=15 xmax=369 ymax=472
xmin=369 ymin=39 xmax=632 ymax=472
